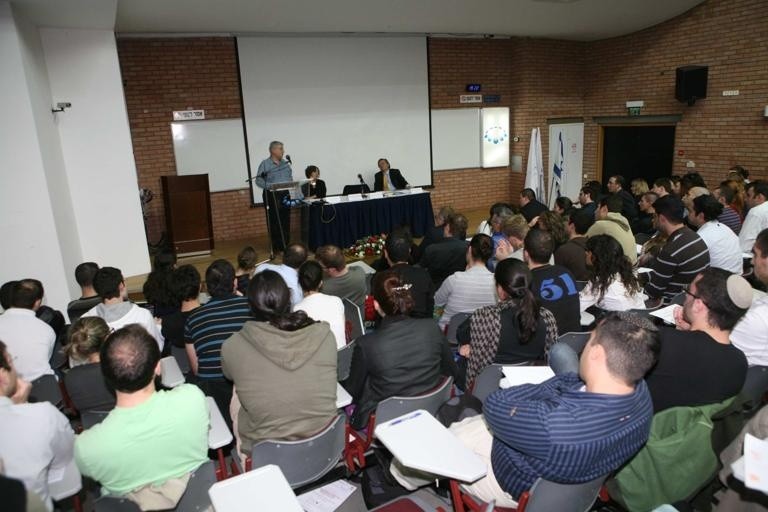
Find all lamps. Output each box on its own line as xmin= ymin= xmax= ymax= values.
xmin=51 ymin=103 xmax=71 ymax=112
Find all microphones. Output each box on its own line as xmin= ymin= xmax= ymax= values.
xmin=358 ymin=174 xmax=364 ymax=183
xmin=286 ymin=155 xmax=292 ymax=164
xmin=317 ymin=179 xmax=325 ymax=186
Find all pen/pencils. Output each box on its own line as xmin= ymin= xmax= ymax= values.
xmin=391 ymin=412 xmax=421 ymax=425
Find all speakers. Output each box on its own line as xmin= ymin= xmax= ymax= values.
xmin=675 ymin=65 xmax=708 ymax=100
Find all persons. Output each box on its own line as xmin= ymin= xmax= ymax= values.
xmin=256 ymin=141 xmax=294 ymax=255
xmin=0 ymin=160 xmax=768 ymax=511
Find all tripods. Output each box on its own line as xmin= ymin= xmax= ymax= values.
xmin=245 ymin=161 xmax=288 ymax=267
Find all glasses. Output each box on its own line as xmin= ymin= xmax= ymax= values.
xmin=683 ymin=285 xmax=701 ymax=299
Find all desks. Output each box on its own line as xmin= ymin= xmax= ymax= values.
xmin=301 ymin=189 xmax=435 ymax=256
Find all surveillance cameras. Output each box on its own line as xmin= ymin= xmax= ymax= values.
xmin=57 ymin=103 xmax=71 ymax=108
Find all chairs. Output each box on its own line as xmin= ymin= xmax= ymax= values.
xmin=343 ymin=184 xmax=370 ymax=196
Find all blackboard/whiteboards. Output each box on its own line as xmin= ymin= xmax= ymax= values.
xmin=168 ymin=107 xmax=482 ymax=194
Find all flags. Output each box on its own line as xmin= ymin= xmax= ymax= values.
xmin=523 ymin=126 xmax=565 ymax=210
